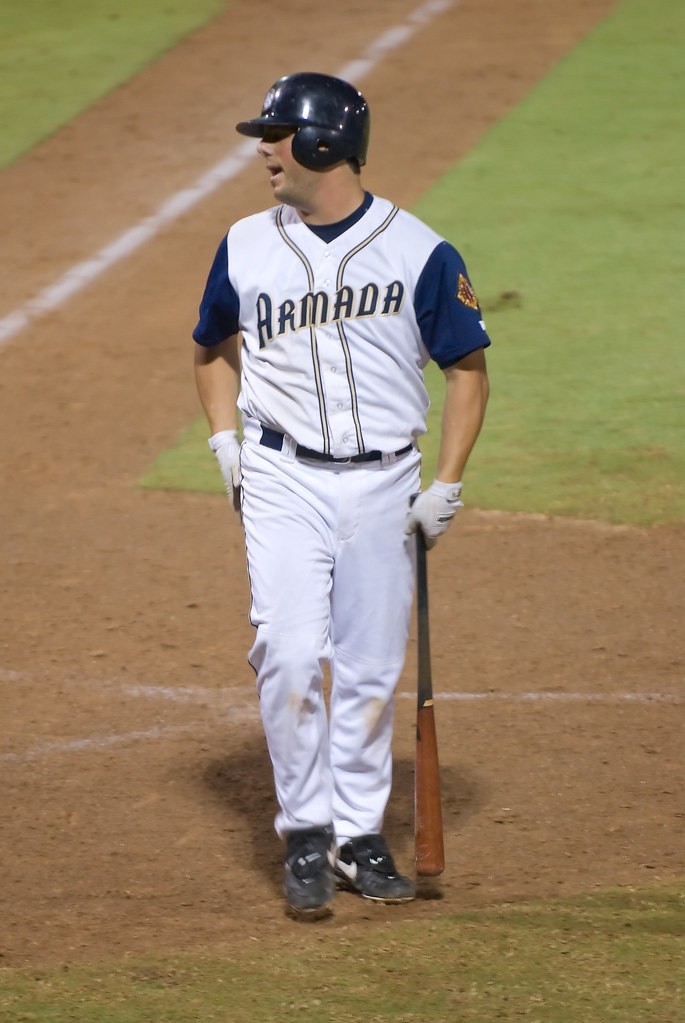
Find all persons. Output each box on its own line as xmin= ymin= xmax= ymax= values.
xmin=191 ymin=70 xmax=493 ymax=921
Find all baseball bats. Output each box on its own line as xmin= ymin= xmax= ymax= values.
xmin=409 ymin=489 xmax=446 ymax=878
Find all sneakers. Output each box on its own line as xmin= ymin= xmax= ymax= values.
xmin=326 ymin=834 xmax=418 ymax=906
xmin=281 ymin=830 xmax=334 ymax=921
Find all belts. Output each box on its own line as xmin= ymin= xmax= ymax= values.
xmin=260 ymin=423 xmax=412 ymax=465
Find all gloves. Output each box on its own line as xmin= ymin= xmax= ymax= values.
xmin=405 ymin=479 xmax=467 ymax=551
xmin=206 ymin=429 xmax=246 ymax=526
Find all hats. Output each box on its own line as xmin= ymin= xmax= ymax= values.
xmin=235 ymin=72 xmax=372 ymax=172
